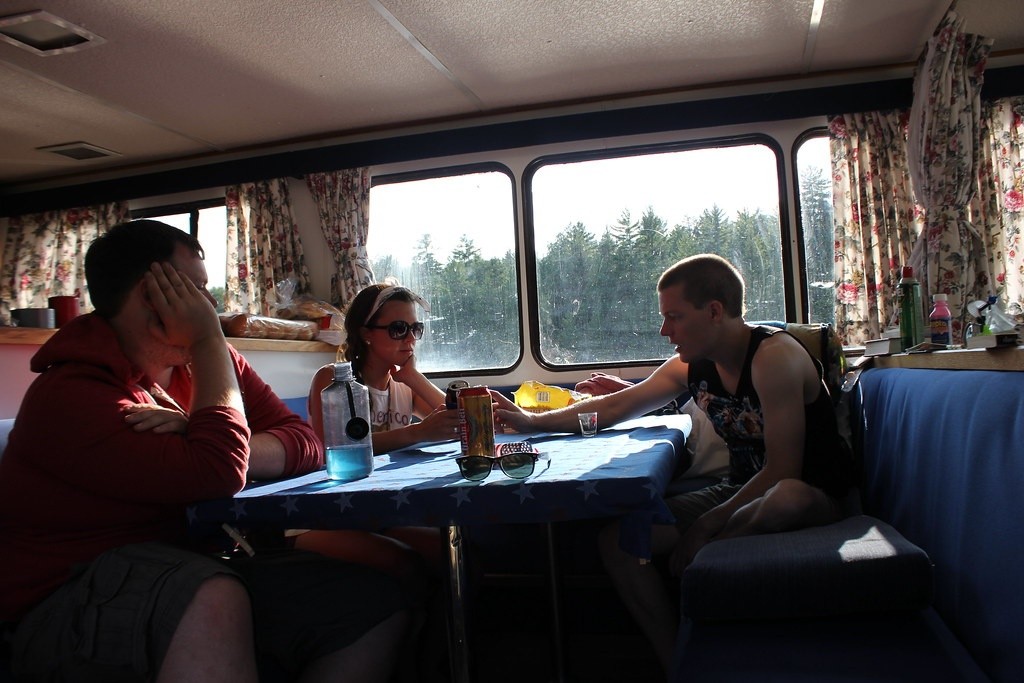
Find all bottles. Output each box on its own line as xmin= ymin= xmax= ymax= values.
xmin=929 ymin=293 xmax=952 ymax=344
xmin=321 ymin=362 xmax=372 ymax=481
xmin=897 ymin=268 xmax=924 ymax=350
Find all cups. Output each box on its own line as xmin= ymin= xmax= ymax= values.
xmin=578 ymin=412 xmax=598 ymax=437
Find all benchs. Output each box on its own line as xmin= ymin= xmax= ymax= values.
xmin=0 ymin=396 xmax=309 ymax=456
xmin=687 ymin=345 xmax=1024 ymax=683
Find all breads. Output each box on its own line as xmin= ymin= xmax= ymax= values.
xmin=220 ymin=314 xmax=318 ymax=339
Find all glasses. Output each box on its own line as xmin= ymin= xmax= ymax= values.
xmin=455 ymin=448 xmax=551 ymax=481
xmin=368 ymin=321 xmax=425 ymax=340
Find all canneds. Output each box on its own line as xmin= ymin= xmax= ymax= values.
xmin=445 ymin=380 xmax=468 ymax=410
xmin=458 ymin=386 xmax=496 ymax=469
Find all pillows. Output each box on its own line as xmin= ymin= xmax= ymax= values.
xmin=683 ymin=513 xmax=942 ymax=627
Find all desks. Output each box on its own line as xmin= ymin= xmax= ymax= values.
xmin=185 ymin=413 xmax=693 ymax=683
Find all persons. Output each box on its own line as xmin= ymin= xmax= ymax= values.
xmin=243 ymin=285 xmax=461 ymax=683
xmin=488 ymin=252 xmax=848 ymax=683
xmin=0 ymin=221 xmax=409 ymax=683
